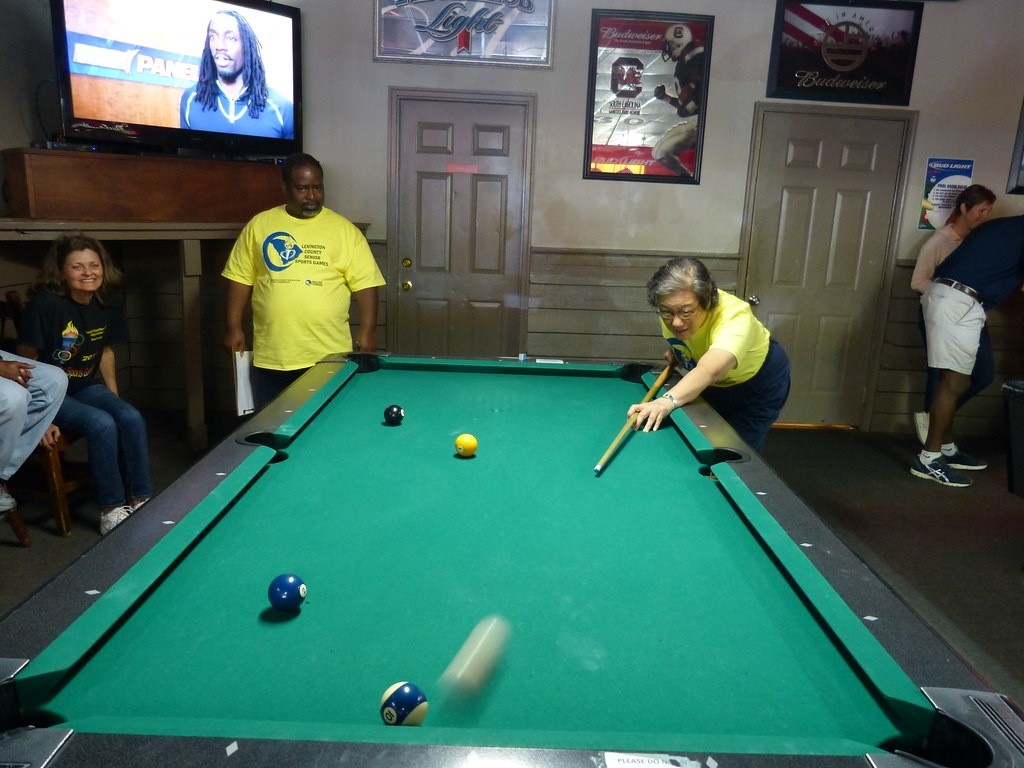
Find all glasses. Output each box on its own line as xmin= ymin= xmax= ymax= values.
xmin=657 ymin=299 xmax=701 ymax=320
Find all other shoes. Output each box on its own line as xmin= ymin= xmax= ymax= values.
xmin=913 ymin=412 xmax=930 ymax=445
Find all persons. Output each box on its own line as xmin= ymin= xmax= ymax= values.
xmin=219 ymin=152 xmax=386 ymax=412
xmin=15 ymin=233 xmax=154 ymax=534
xmin=911 ymin=214 xmax=1024 ymax=488
xmin=627 ymin=256 xmax=790 ymax=454
xmin=179 ymin=11 xmax=293 ymax=138
xmin=910 ymin=184 xmax=997 ymax=444
xmin=0 ymin=349 xmax=68 ymax=513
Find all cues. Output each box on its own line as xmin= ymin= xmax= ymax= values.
xmin=593 ymin=364 xmax=675 ymax=474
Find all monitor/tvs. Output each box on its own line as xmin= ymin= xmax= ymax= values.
xmin=49 ymin=0 xmax=303 ymax=161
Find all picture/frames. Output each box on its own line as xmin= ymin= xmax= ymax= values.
xmin=582 ymin=7 xmax=716 ymax=186
xmin=372 ymin=0 xmax=556 ymax=71
xmin=766 ymin=0 xmax=924 ymax=107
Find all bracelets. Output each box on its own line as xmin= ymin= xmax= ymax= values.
xmin=662 ymin=394 xmax=677 ymax=407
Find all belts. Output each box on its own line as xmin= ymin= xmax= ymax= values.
xmin=938 ymin=278 xmax=981 ymax=303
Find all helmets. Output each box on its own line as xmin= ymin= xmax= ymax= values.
xmin=663 ymin=24 xmax=693 ymax=58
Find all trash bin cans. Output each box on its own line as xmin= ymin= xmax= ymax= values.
xmin=1000 ymin=380 xmax=1024 ymax=495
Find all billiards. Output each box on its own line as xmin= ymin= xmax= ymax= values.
xmin=268 ymin=572 xmax=307 ymax=611
xmin=440 ymin=614 xmax=512 ymax=695
xmin=455 ymin=433 xmax=478 ymax=457
xmin=384 ymin=405 xmax=404 ymax=424
xmin=380 ymin=681 xmax=429 ymax=726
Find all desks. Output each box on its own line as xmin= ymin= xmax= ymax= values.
xmin=0 ymin=349 xmax=1024 ymax=768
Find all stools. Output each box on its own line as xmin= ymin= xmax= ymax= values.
xmin=25 ymin=423 xmax=131 ymax=538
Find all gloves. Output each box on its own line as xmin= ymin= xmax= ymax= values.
xmin=678 ymin=105 xmax=690 ymax=118
xmin=654 ymin=84 xmax=665 ymax=99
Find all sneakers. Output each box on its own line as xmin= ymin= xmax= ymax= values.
xmin=100 ymin=505 xmax=133 ymax=535
xmin=943 ymin=449 xmax=988 ymax=470
xmin=910 ymin=455 xmax=973 ymax=487
xmin=0 ymin=484 xmax=17 ymax=512
